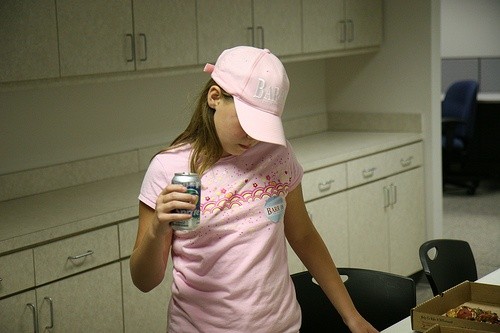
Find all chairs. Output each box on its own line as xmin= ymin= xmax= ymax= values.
xmin=440 ymin=79 xmax=482 ymax=195
xmin=290 ymin=267 xmax=417 ymax=333
xmin=419 ymin=240 xmax=477 ymax=296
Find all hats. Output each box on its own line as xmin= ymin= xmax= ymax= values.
xmin=203 ymin=46 xmax=290 ymax=148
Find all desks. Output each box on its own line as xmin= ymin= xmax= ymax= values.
xmin=441 ymin=92 xmax=500 ymax=105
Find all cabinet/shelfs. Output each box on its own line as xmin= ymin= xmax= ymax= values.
xmin=0 ymin=130 xmax=431 ymax=333
xmin=0 ymin=0 xmax=384 ymax=91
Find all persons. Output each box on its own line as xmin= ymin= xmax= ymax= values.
xmin=130 ymin=45 xmax=378 ymax=333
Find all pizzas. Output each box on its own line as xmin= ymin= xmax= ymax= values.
xmin=446 ymin=306 xmax=500 ymax=324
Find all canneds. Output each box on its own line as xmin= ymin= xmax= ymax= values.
xmin=170 ymin=172 xmax=201 ymax=230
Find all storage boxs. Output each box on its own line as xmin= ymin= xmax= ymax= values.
xmin=410 ymin=280 xmax=500 ymax=333
